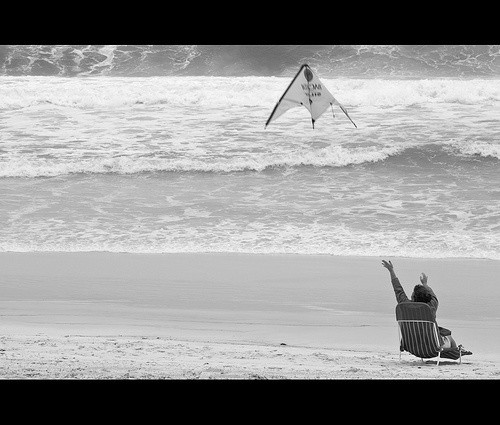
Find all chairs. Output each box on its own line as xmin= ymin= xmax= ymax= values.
xmin=395 ymin=302 xmax=474 ymax=364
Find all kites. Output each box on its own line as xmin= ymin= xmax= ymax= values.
xmin=265 ymin=64 xmax=357 ymax=129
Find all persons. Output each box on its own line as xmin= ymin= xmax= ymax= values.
xmin=382 ymin=260 xmax=457 ymax=349
